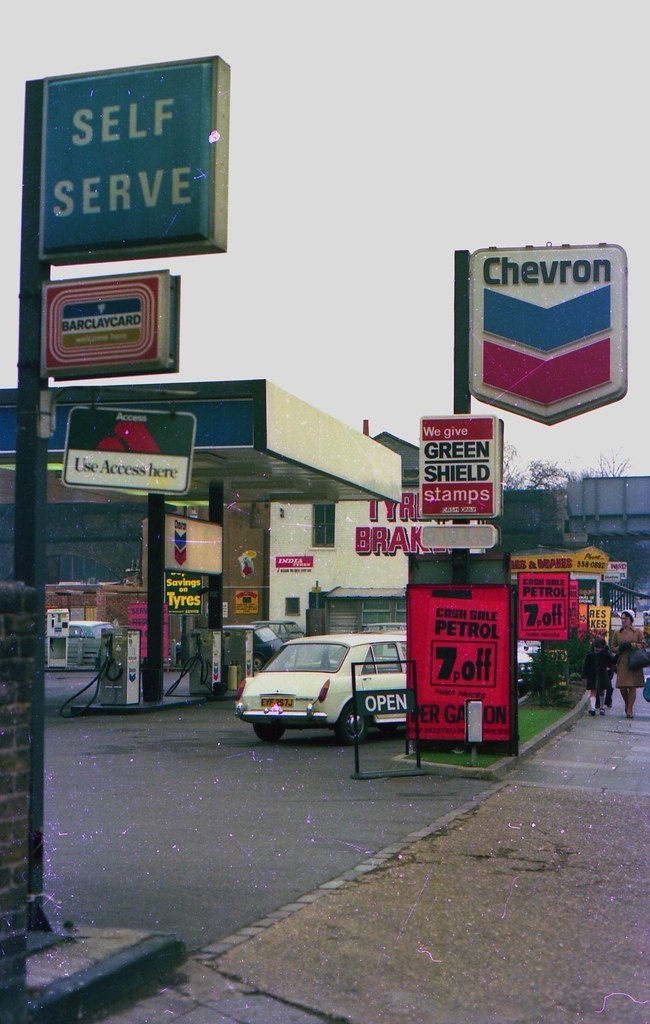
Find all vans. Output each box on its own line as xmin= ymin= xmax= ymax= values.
xmin=69 ymin=620 xmax=114 ymax=665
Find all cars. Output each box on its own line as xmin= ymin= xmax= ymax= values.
xmin=517 ymin=641 xmax=542 ymax=696
xmin=176 ymin=621 xmax=407 ymax=672
xmin=234 ymin=632 xmax=407 ymax=745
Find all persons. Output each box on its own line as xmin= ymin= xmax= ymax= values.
xmin=581 ymin=611 xmax=650 ymax=720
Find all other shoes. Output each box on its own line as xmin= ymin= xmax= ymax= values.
xmin=595 ymin=705 xmax=600 ymax=708
xmin=605 ymin=703 xmax=612 ymax=707
xmin=627 ymin=714 xmax=633 ymax=718
xmin=599 ymin=709 xmax=605 ymax=714
xmin=589 ymin=708 xmax=596 ymax=715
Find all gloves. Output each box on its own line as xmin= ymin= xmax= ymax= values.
xmin=619 ymin=642 xmax=631 ymax=651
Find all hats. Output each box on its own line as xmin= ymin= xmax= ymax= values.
xmin=622 ymin=610 xmax=636 ymax=623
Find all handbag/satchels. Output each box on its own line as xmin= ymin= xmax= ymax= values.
xmin=629 ymin=645 xmax=650 ymax=669
